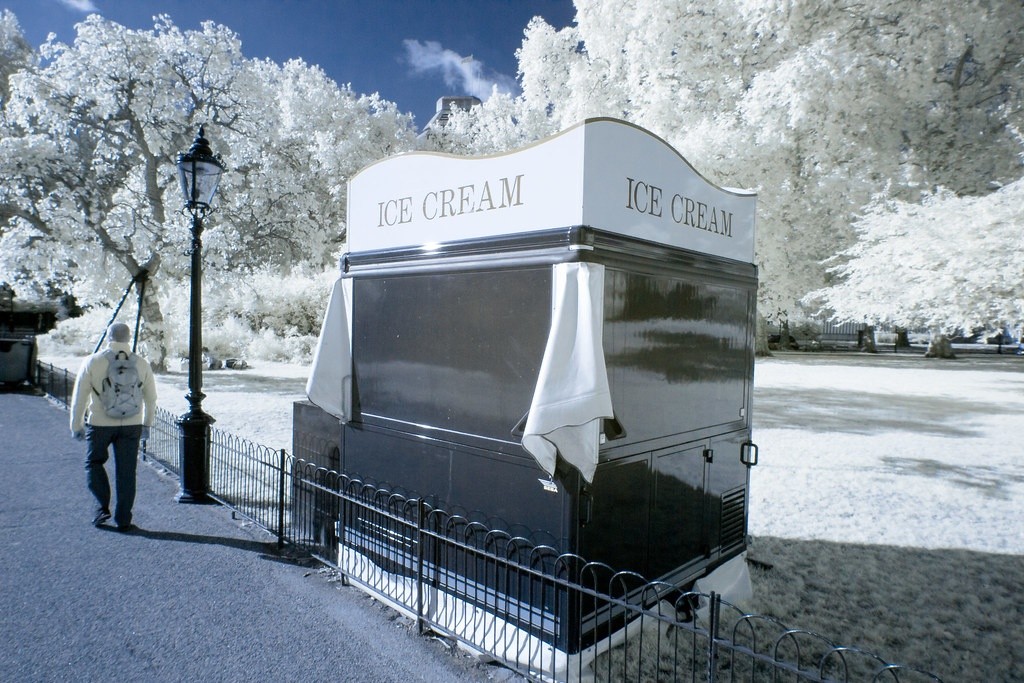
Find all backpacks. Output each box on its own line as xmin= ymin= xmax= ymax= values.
xmin=102 ymin=351 xmax=144 ymax=420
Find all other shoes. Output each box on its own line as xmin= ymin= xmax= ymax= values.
xmin=91 ymin=511 xmax=111 ymax=527
xmin=116 ymin=522 xmax=132 ymax=531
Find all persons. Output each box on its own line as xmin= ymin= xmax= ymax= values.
xmin=70 ymin=324 xmax=157 ymax=532
xmin=778 ymin=324 xmax=790 ymax=351
xmin=202 ymin=346 xmax=215 ymax=370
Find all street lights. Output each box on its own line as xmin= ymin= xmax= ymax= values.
xmin=172 ymin=124 xmax=221 ymax=505
